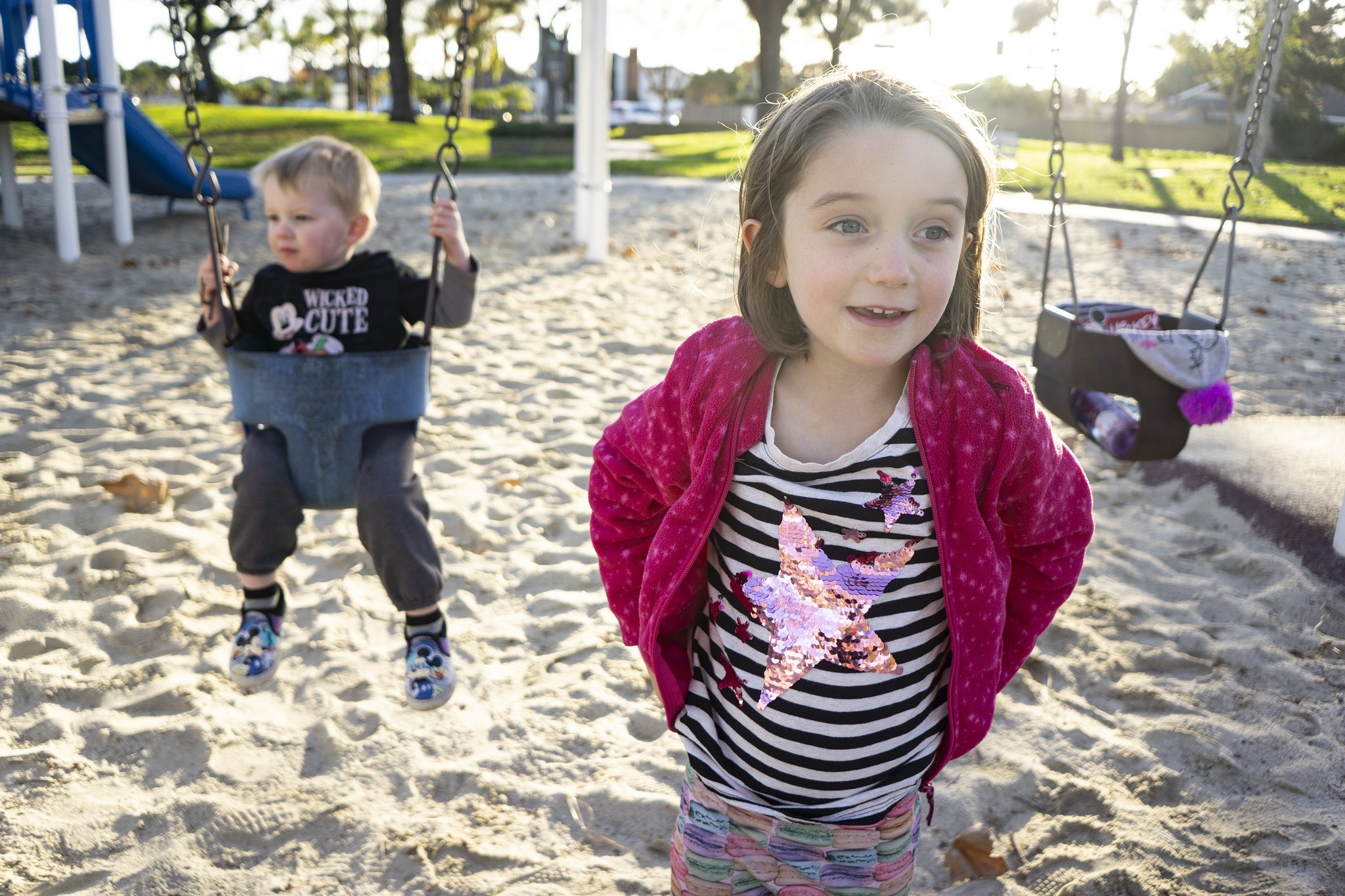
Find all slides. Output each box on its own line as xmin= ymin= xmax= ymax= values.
xmin=0 ymin=1 xmax=255 ymax=221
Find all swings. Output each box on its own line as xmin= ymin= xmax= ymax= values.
xmin=160 ymin=3 xmax=485 ymax=514
xmin=1028 ymin=0 xmax=1293 ymax=464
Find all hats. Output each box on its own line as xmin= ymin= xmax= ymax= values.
xmin=1115 ymin=328 xmax=1236 ymax=425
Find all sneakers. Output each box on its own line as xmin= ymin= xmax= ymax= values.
xmin=230 ymin=576 xmax=287 ymax=690
xmin=401 ymin=623 xmax=454 ymax=710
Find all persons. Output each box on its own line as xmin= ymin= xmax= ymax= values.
xmin=197 ymin=135 xmax=478 ymax=711
xmin=587 ymin=68 xmax=1096 ymax=894
